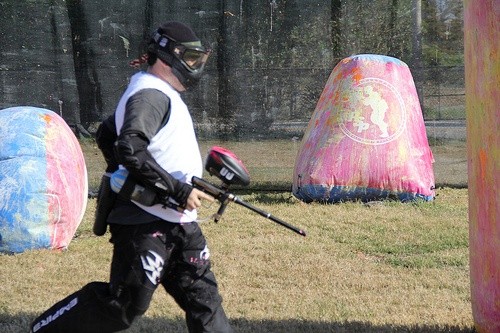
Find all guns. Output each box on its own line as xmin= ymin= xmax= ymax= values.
xmin=189 ymin=145 xmax=307 ymax=238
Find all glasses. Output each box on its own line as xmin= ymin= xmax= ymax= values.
xmin=179 ymin=46 xmax=208 ymax=73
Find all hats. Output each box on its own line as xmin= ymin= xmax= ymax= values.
xmin=149 ymin=21 xmax=209 ymax=92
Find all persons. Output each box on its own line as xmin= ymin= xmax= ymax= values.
xmin=28 ymin=22 xmax=233 ymax=333
